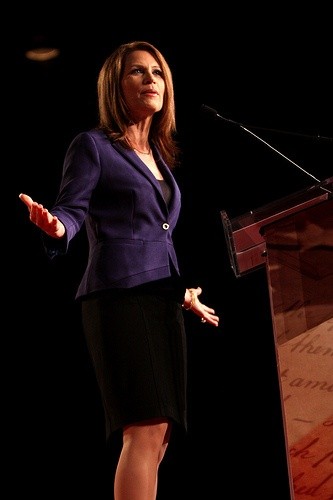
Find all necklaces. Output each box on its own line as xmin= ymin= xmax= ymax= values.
xmin=134 ymin=146 xmax=152 ymax=155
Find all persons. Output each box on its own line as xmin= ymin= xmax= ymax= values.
xmin=19 ymin=42 xmax=219 ymax=500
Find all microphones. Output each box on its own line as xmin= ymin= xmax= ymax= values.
xmin=200 ymin=103 xmax=333 ymax=146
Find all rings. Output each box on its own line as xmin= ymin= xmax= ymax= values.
xmin=201 ymin=318 xmax=206 ymax=323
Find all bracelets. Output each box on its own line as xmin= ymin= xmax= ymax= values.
xmin=182 ymin=288 xmax=196 ymax=311
